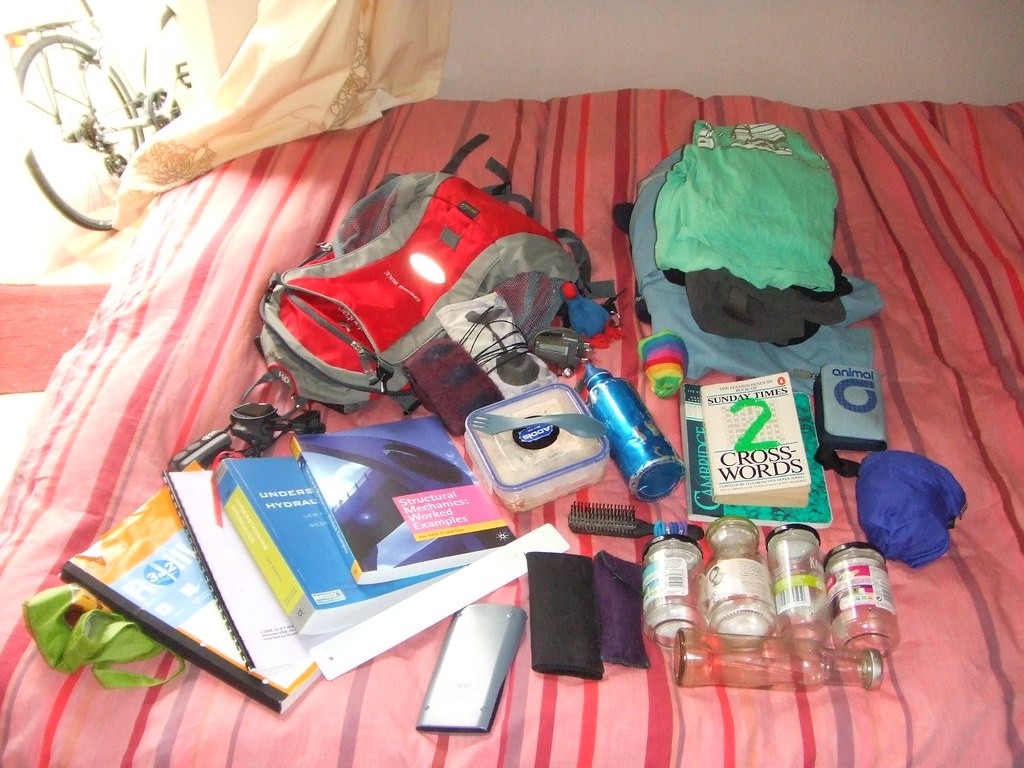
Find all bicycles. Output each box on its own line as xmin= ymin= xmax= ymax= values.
xmin=3 ymin=0 xmax=192 ymax=233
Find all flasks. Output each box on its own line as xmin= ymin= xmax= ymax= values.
xmin=585 ymin=364 xmax=686 ymax=501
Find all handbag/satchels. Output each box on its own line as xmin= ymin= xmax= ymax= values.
xmin=23 ymin=582 xmax=185 ymax=688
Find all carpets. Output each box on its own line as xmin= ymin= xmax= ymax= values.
xmin=0 ymin=284 xmax=116 ymax=395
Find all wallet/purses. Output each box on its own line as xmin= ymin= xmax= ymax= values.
xmin=526 ymin=551 xmax=605 ymax=681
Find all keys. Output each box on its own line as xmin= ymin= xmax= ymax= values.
xmin=602 ymin=286 xmax=629 ymax=306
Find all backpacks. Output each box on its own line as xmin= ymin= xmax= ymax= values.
xmin=255 ymin=170 xmax=591 ymax=415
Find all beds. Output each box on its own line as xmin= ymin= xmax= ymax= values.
xmin=0 ymin=90 xmax=1022 ymax=767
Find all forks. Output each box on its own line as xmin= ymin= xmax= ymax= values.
xmin=471 ymin=413 xmax=606 ymax=439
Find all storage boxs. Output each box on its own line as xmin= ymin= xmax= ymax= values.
xmin=467 ymin=384 xmax=608 ymax=508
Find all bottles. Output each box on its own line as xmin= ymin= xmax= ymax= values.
xmin=766 ymin=523 xmax=831 ymax=640
xmin=705 ymin=515 xmax=776 ymax=638
xmin=640 ymin=534 xmax=707 ymax=651
xmin=674 ymin=629 xmax=883 ymax=694
xmin=824 ymin=542 xmax=899 ymax=658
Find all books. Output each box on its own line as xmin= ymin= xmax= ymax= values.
xmin=698 ymin=372 xmax=811 ymax=509
xmin=288 ymin=414 xmax=519 ymax=585
xmin=678 ymin=382 xmax=834 ymax=531
xmin=211 ymin=456 xmax=469 ymax=636
xmin=63 ymin=459 xmax=324 ymax=716
xmin=163 ymin=469 xmax=320 ymax=667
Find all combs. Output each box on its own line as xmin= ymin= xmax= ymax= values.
xmin=568 ymin=500 xmax=705 ymax=543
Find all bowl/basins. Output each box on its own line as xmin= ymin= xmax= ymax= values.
xmin=466 ymin=382 xmax=610 ymax=512
